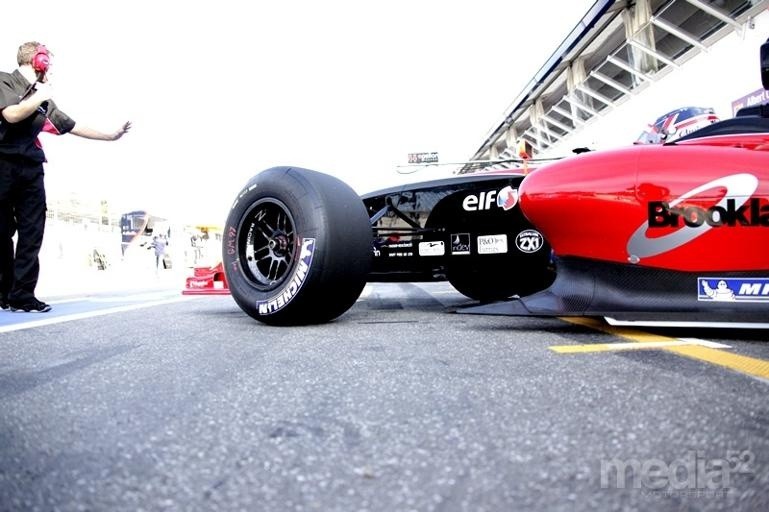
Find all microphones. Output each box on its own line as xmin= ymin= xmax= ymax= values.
xmin=44 ymin=71 xmax=52 ymax=87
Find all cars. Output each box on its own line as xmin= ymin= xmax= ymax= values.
xmin=222 ymin=37 xmax=768 ymax=329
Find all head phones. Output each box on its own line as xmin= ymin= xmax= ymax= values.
xmin=32 ymin=43 xmax=51 ymax=72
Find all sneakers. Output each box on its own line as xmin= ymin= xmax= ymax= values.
xmin=0 ymin=297 xmax=52 ymax=313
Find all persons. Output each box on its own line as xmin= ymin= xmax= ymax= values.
xmin=152 ymin=233 xmax=170 ymax=271
xmin=0 ymin=40 xmax=133 ymax=314
xmin=188 ymin=233 xmax=210 ymax=265
